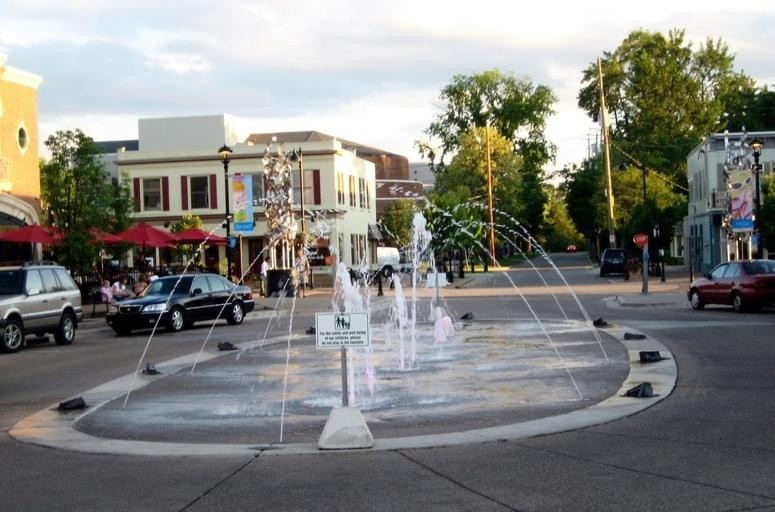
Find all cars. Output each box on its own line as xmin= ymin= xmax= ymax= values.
xmin=103 ymin=271 xmax=256 ymax=338
xmin=685 ymin=259 xmax=775 ymax=313
xmin=599 ymin=247 xmax=630 ymax=279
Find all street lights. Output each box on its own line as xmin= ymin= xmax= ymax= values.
xmin=216 ymin=142 xmax=235 ymax=285
xmin=746 ymin=133 xmax=764 ymax=259
xmin=270 ymin=147 xmax=306 ymax=270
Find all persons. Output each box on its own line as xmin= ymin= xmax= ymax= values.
xmin=101 ymin=274 xmax=159 ymax=302
xmin=294 ymin=249 xmax=311 ymax=298
xmin=260 ymin=256 xmax=272 ymax=296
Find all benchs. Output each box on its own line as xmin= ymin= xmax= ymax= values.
xmin=90 ymin=286 xmax=112 ymax=317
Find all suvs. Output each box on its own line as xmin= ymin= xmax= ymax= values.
xmin=0 ymin=260 xmax=83 ymax=356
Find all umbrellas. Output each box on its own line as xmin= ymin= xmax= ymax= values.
xmin=0 ymin=221 xmax=227 ymax=273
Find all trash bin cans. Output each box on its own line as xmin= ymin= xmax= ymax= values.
xmin=267 ymin=269 xmax=298 ymax=297
